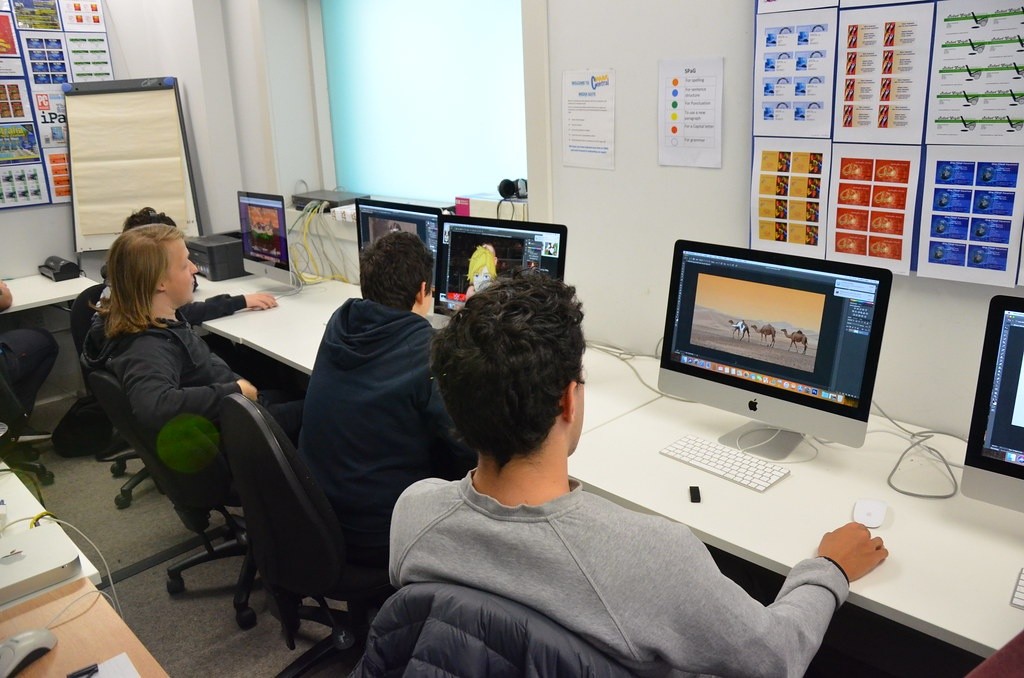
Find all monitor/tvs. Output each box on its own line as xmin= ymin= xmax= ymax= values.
xmin=433 ymin=215 xmax=568 ymax=316
xmin=354 ymin=198 xmax=444 ymax=298
xmin=236 ymin=191 xmax=302 ymax=296
xmin=960 ymin=295 xmax=1024 ymax=513
xmin=658 ymin=239 xmax=894 ymax=460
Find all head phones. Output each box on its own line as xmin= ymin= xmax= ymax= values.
xmin=498 ymin=179 xmax=528 ymax=200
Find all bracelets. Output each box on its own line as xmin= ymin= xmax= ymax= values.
xmin=815 ymin=556 xmax=850 ymax=588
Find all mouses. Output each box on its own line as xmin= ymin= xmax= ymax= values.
xmin=0 ymin=629 xmax=58 ymax=678
xmin=853 ymin=497 xmax=888 ymax=528
xmin=252 ymin=303 xmax=278 ymax=310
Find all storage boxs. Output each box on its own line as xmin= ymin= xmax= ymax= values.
xmin=454 ymin=193 xmax=529 ymax=222
xmin=330 ymin=203 xmax=357 ymax=223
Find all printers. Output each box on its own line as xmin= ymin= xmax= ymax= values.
xmin=185 ymin=230 xmax=253 ymax=281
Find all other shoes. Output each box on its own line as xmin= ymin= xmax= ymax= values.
xmin=17 ymin=425 xmax=53 ymax=442
xmin=97 ymin=438 xmax=135 ymax=462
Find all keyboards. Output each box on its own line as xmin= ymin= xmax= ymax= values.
xmin=659 ymin=434 xmax=791 ymax=493
xmin=1009 ymin=568 xmax=1024 ymax=611
xmin=229 ymin=288 xmax=249 ymax=298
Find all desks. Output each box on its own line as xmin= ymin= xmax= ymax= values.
xmin=0 ymin=577 xmax=171 ymax=678
xmin=189 ymin=271 xmax=1023 ymax=660
xmin=0 ymin=274 xmax=100 ymax=315
xmin=0 ymin=460 xmax=102 ymax=612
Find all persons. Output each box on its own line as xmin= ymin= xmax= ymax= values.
xmin=389 ymin=266 xmax=889 ymax=678
xmin=0 ymin=289 xmax=53 ymax=446
xmin=82 ymin=223 xmax=305 ymax=479
xmin=122 ymin=208 xmax=276 ymax=324
xmin=298 ymin=231 xmax=480 ymax=563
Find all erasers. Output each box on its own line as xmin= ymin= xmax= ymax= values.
xmin=690 ymin=486 xmax=701 ymax=503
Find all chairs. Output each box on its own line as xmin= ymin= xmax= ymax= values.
xmin=0 ymin=343 xmax=54 ymax=486
xmin=69 ymin=283 xmax=388 ymax=652
xmin=346 ymin=582 xmax=633 ymax=678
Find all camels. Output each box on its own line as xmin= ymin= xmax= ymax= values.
xmin=751 ymin=324 xmax=776 ymax=348
xmin=729 ymin=320 xmax=750 ymax=342
xmin=781 ymin=329 xmax=808 ymax=354
